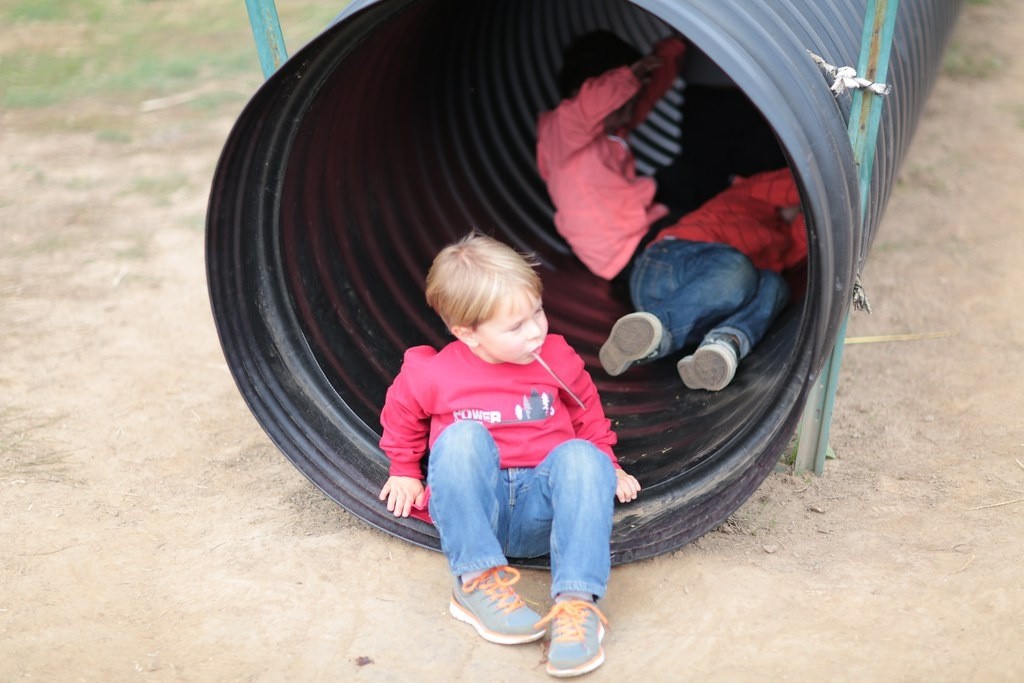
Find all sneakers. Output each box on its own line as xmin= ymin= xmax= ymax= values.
xmin=534 ymin=593 xmax=611 ymax=678
xmin=450 ymin=566 xmax=547 ymax=645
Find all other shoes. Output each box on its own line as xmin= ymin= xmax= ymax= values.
xmin=599 ymin=310 xmax=663 ymax=376
xmin=677 ymin=338 xmax=738 ymax=390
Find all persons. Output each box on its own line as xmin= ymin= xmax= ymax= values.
xmin=534 ymin=29 xmax=791 ymax=283
xmin=600 ymin=167 xmax=810 ymax=392
xmin=379 ymin=234 xmax=640 ymax=678
